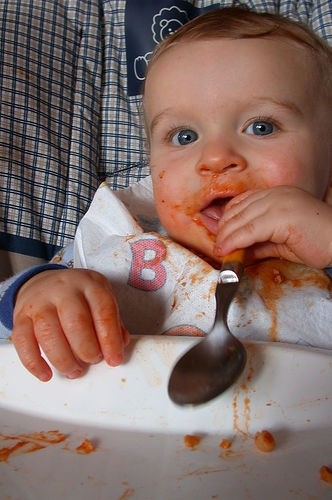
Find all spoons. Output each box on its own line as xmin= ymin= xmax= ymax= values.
xmin=168 ymin=248 xmax=247 ymax=408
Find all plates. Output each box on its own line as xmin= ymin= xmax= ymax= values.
xmin=1 ymin=334 xmax=331 ymax=499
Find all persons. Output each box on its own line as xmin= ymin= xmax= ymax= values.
xmin=1 ymin=6 xmax=332 ymax=384
